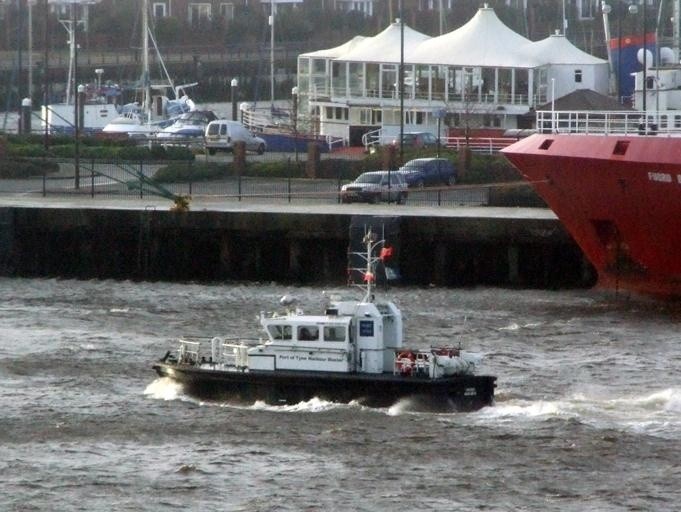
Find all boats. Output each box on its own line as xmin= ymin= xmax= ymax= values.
xmin=103 ymin=0 xmax=219 ymax=140
xmin=151 ymin=222 xmax=497 ymax=413
xmin=498 ymin=110 xmax=681 ymax=303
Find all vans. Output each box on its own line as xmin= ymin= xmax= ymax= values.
xmin=205 ymin=120 xmax=266 ymax=155
xmin=399 ymin=158 xmax=457 ymax=187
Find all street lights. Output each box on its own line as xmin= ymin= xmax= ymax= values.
xmin=395 ymin=11 xmax=404 ymax=158
xmin=95 ymin=69 xmax=104 ymax=89
xmin=291 ymin=87 xmax=298 ymax=128
xmin=78 ymin=85 xmax=85 ymax=128
xmin=21 ymin=98 xmax=32 ymax=134
xmin=231 ymin=79 xmax=237 ymax=121
xmin=602 ymin=2 xmax=638 ymax=103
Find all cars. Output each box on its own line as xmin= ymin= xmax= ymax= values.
xmin=393 ymin=132 xmax=437 ymax=145
xmin=341 ymin=170 xmax=408 ymax=204
